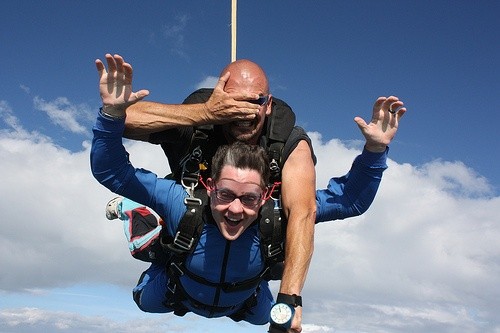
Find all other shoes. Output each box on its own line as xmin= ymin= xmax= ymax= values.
xmin=105 ymin=197 xmax=122 ymax=221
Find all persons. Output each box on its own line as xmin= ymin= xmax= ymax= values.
xmin=123 ymin=59 xmax=319 ymax=333
xmin=88 ymin=53 xmax=407 ymax=327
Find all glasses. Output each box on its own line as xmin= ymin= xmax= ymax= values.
xmin=241 ymin=96 xmax=268 ymax=107
xmin=214 ymin=184 xmax=263 ymax=205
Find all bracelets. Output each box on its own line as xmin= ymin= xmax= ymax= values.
xmin=99 ymin=107 xmax=129 ymax=124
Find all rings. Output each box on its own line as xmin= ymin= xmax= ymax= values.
xmin=388 ymin=108 xmax=397 ymax=114
